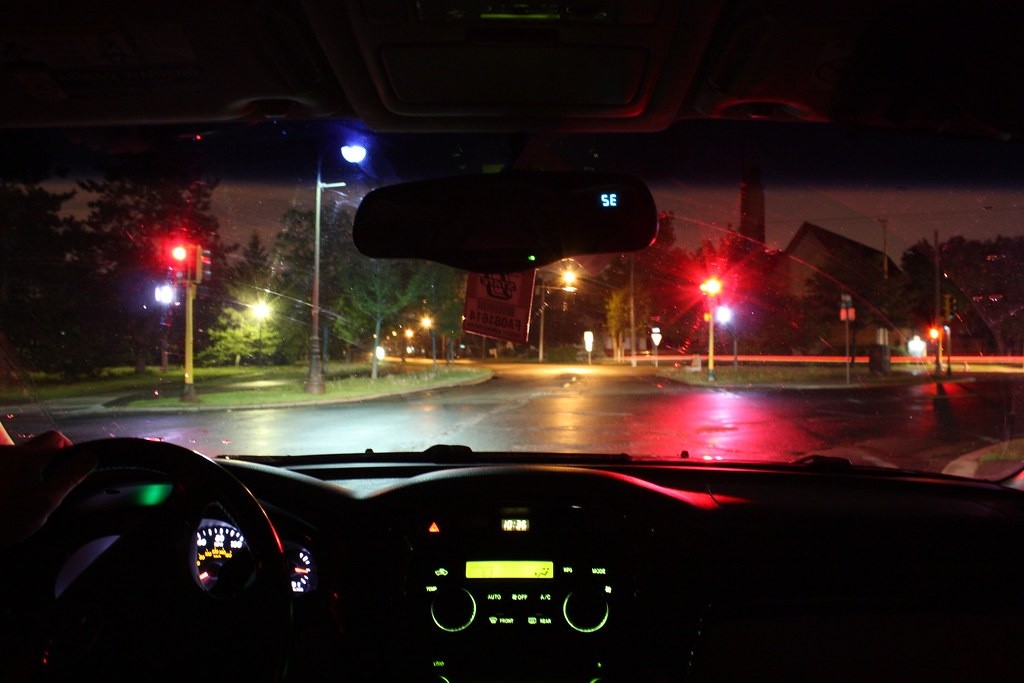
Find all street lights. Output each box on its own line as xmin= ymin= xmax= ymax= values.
xmin=539 ymin=271 xmax=576 ymax=362
xmin=698 ymin=275 xmax=724 ymax=383
xmin=303 ymin=125 xmax=369 ymax=396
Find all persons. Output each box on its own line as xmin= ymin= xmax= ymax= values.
xmin=0 ymin=431 xmax=98 ymax=546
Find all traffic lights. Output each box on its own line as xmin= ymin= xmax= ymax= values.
xmin=168 ymin=240 xmax=192 ymax=267
xmin=194 ymin=243 xmax=213 ymax=283
